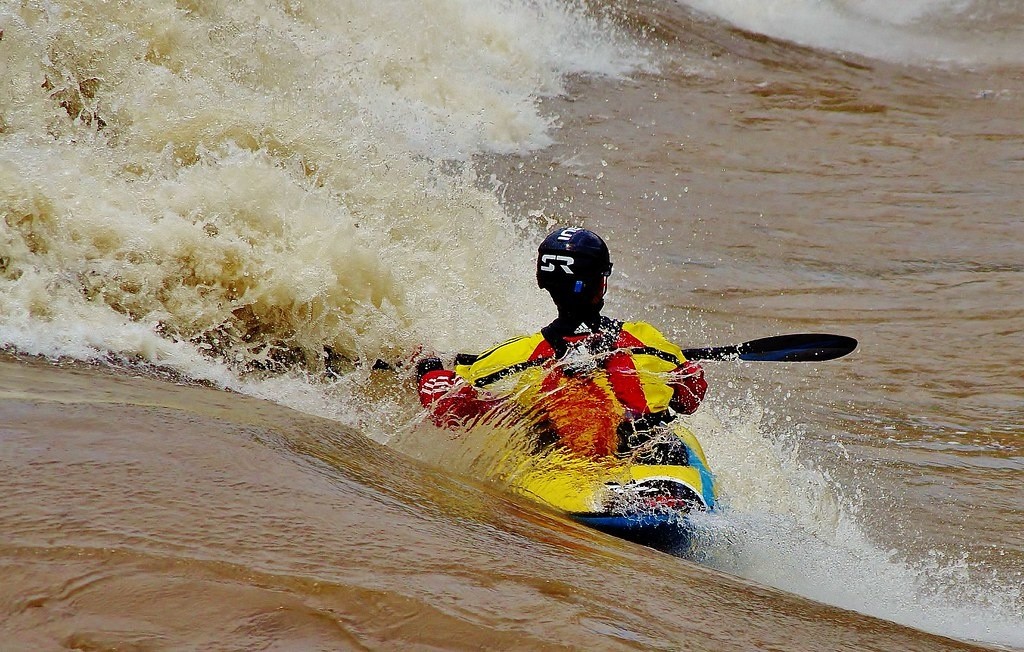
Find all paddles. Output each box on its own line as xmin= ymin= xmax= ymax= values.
xmin=321 ymin=328 xmax=859 ymax=381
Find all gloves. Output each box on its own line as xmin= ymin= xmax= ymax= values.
xmin=418 ymin=358 xmax=444 ymax=382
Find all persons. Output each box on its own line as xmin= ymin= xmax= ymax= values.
xmin=417 ymin=228 xmax=707 ymax=466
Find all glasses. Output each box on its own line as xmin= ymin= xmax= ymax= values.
xmin=604 ymin=261 xmax=614 ymax=275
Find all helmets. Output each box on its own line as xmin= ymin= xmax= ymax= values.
xmin=535 ymin=225 xmax=610 ymax=289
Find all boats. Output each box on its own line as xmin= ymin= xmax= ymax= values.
xmin=477 ymin=424 xmax=715 ymax=534
